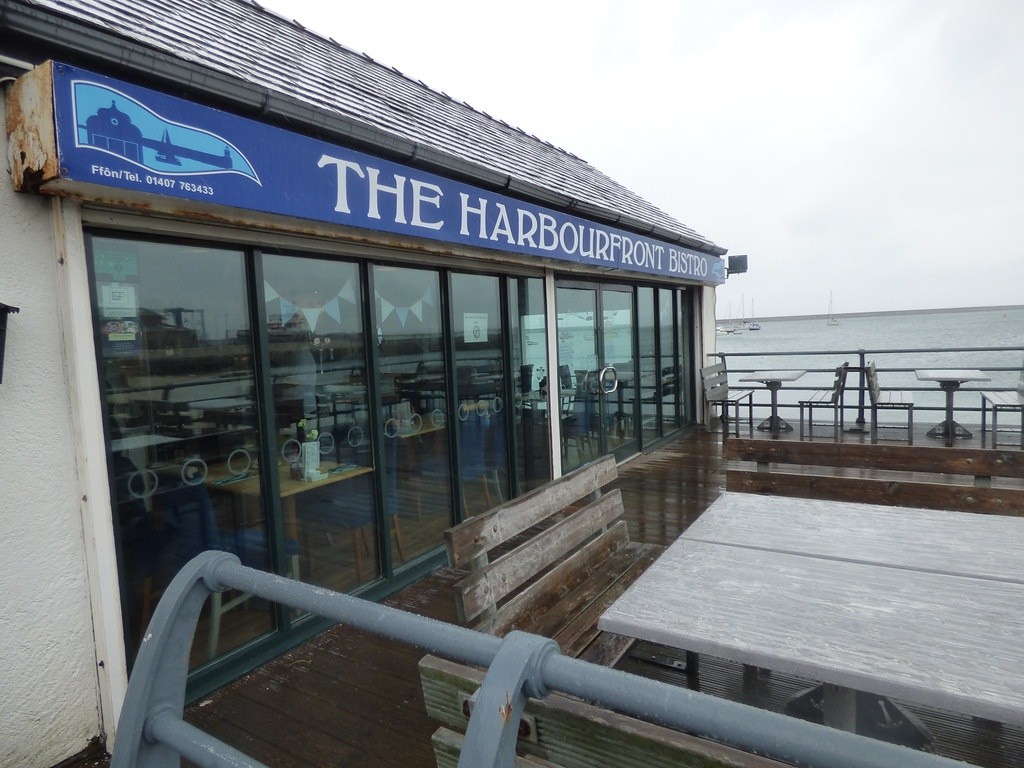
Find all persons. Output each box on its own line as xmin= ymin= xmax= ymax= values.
xmin=267 ymin=321 xmax=318 ymax=429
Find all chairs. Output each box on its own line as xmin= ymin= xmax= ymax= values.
xmin=107 ymin=364 xmax=684 ymax=663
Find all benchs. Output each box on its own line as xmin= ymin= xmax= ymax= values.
xmin=725 ymin=437 xmax=1024 ymax=517
xmin=864 ymin=360 xmax=914 ymax=445
xmin=980 ymin=359 xmax=1024 ymax=451
xmin=417 ymin=655 xmax=796 ymax=768
xmin=798 ymin=361 xmax=848 ymax=443
xmin=443 ymin=453 xmax=700 ymax=703
xmin=699 ymin=363 xmax=754 ymax=439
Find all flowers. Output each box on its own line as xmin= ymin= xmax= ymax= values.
xmin=298 ymin=418 xmax=318 ymax=443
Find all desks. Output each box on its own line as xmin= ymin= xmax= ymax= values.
xmin=517 ymin=390 xmax=575 ymax=479
xmin=915 ymin=370 xmax=991 ymax=446
xmin=738 ymin=371 xmax=807 ymax=439
xmin=396 ymin=412 xmax=447 ymax=513
xmin=597 ymin=491 xmax=1024 ymax=745
xmin=584 ymin=372 xmax=654 ymax=438
xmin=196 ymin=457 xmax=373 ymax=540
